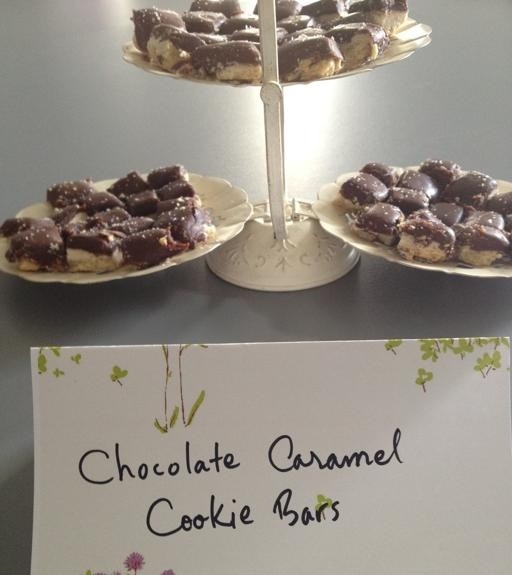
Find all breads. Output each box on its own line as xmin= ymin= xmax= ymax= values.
xmin=132 ymin=0 xmax=409 ymax=82
xmin=0 ymin=163 xmax=215 ymax=274
xmin=338 ymin=157 xmax=511 ymax=268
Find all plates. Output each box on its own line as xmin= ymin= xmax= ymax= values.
xmin=0 ymin=170 xmax=255 ymax=285
xmin=119 ymin=17 xmax=435 ymax=86
xmin=310 ymin=165 xmax=512 ymax=281
